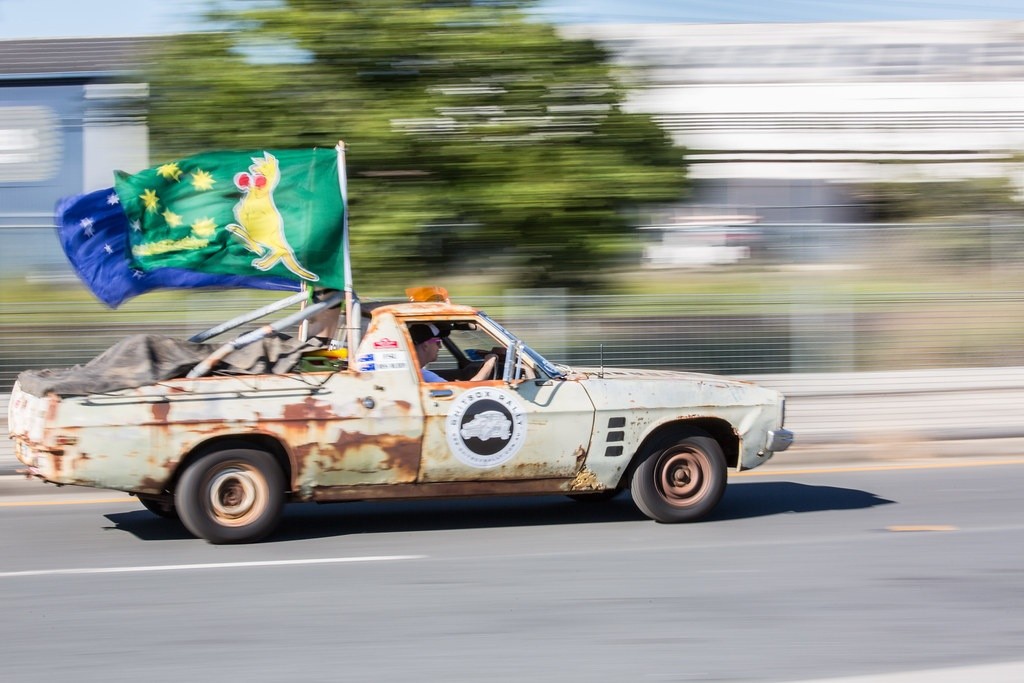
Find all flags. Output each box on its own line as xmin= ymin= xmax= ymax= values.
xmin=53 ymin=187 xmax=304 ymax=311
xmin=113 ymin=143 xmax=348 ymax=292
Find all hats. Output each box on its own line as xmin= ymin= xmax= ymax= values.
xmin=410 ymin=325 xmax=443 ymax=345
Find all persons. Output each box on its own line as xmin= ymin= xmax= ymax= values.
xmin=408 ymin=322 xmax=499 ymax=383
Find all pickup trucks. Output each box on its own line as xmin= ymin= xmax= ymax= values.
xmin=6 ymin=297 xmax=792 ymax=548
